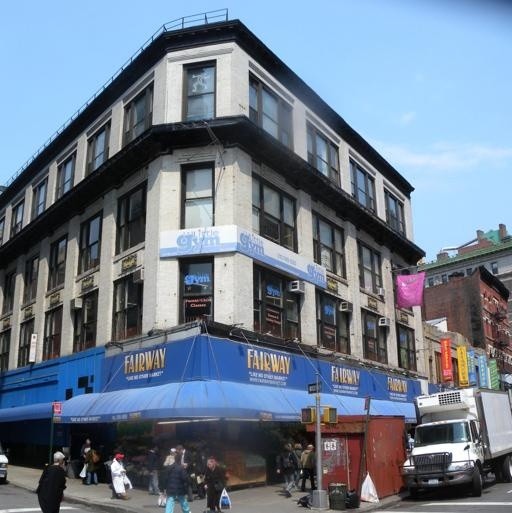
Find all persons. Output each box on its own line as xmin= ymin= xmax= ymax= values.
xmin=36 ymin=449 xmax=70 ymax=512
xmin=275 ymin=439 xmax=319 ymax=498
xmin=76 ymin=434 xmax=232 ymax=512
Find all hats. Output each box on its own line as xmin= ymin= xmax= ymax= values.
xmin=115 ymin=453 xmax=124 ymax=459
xmin=295 ymin=443 xmax=302 ymax=448
xmin=308 ymin=444 xmax=314 ymax=447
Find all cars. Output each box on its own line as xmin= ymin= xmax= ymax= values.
xmin=0 ymin=446 xmax=8 ymax=483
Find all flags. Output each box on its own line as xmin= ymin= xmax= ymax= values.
xmin=440 ymin=337 xmax=501 ymax=390
xmin=395 ymin=271 xmax=428 ymax=309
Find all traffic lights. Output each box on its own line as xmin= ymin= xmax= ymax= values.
xmin=324 ymin=408 xmax=338 ymax=424
xmin=301 ymin=408 xmax=316 ymax=424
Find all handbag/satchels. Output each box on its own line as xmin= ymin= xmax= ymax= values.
xmin=92 ymin=449 xmax=99 ymax=462
xmin=297 ymin=458 xmax=302 ymax=468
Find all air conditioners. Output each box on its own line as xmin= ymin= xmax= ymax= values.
xmin=71 ymin=297 xmax=82 ymax=309
xmin=377 ymin=287 xmax=390 ymax=326
xmin=339 ymin=301 xmax=353 ymax=312
xmin=290 ymin=280 xmax=306 ymax=294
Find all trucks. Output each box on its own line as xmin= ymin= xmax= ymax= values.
xmin=399 ymin=388 xmax=512 ymax=496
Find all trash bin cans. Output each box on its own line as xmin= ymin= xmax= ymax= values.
xmin=329 ymin=483 xmax=346 ymax=510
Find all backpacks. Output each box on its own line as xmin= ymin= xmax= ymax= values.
xmin=281 ymin=450 xmax=292 ymax=468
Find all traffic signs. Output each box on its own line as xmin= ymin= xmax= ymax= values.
xmin=308 ymin=382 xmax=321 ymax=392
